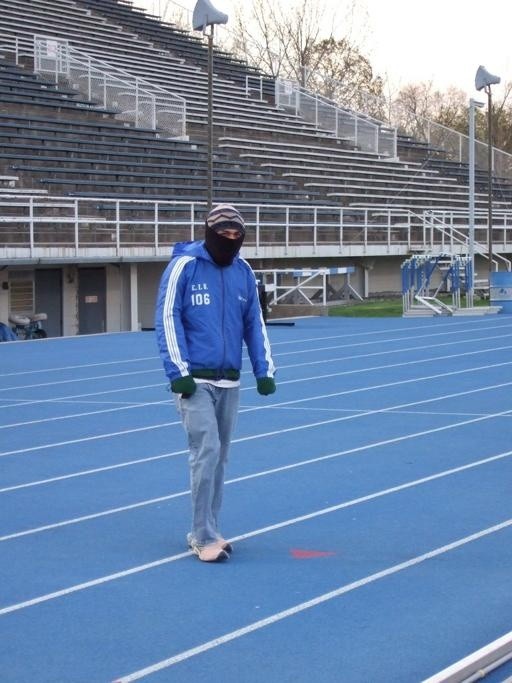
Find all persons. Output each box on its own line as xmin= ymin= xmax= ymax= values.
xmin=155 ymin=202 xmax=277 ymax=562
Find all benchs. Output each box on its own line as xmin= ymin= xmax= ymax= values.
xmin=0 ymin=3 xmax=511 ymax=246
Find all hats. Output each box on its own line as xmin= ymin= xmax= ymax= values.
xmin=206 ymin=204 xmax=246 ymax=234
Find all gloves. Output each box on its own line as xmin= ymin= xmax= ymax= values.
xmin=255 ymin=377 xmax=277 ymax=396
xmin=170 ymin=376 xmax=197 ymax=395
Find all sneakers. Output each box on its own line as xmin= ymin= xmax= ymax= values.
xmin=188 ymin=532 xmax=233 ymax=562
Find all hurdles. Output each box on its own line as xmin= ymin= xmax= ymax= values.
xmin=401 ymin=252 xmax=502 ymax=317
xmin=260 ymin=266 xmax=363 ymax=306
